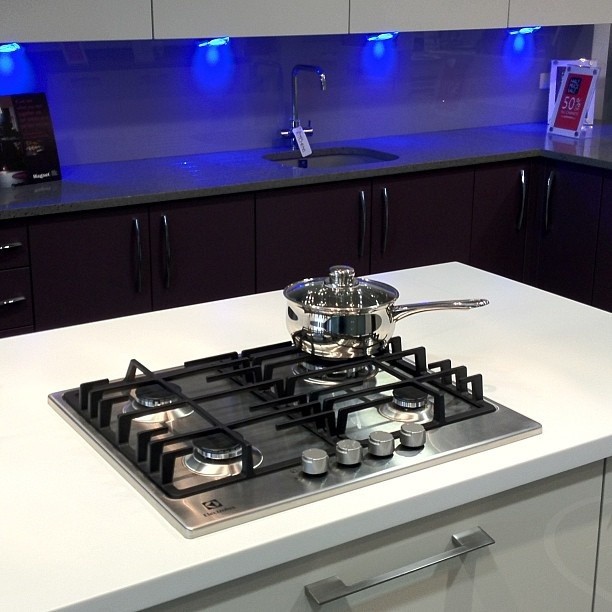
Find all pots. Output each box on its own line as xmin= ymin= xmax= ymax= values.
xmin=282 ymin=264 xmax=489 ymax=358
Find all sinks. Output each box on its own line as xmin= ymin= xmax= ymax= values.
xmin=262 ymin=146 xmax=400 ymax=172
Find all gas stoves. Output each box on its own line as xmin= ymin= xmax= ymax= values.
xmin=47 ymin=336 xmax=542 ymax=539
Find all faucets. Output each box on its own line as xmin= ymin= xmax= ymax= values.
xmin=296 ymin=62 xmax=328 ymax=91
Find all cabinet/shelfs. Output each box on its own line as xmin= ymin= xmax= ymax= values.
xmin=2 ymin=323 xmax=36 ymax=341
xmin=257 ymin=166 xmax=474 ymax=297
xmin=475 ymin=157 xmax=537 ymax=286
xmin=536 ymin=156 xmax=604 ymax=304
xmin=0 ymin=228 xmax=29 ymax=269
xmin=0 ymin=268 xmax=41 ymax=326
xmin=31 ymin=190 xmax=255 ymax=332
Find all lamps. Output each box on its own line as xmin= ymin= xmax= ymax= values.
xmin=198 ymin=36 xmax=231 ymax=48
xmin=0 ymin=43 xmax=22 ymax=54
xmin=368 ymin=33 xmax=397 ymax=45
xmin=508 ymin=27 xmax=543 ymax=38
